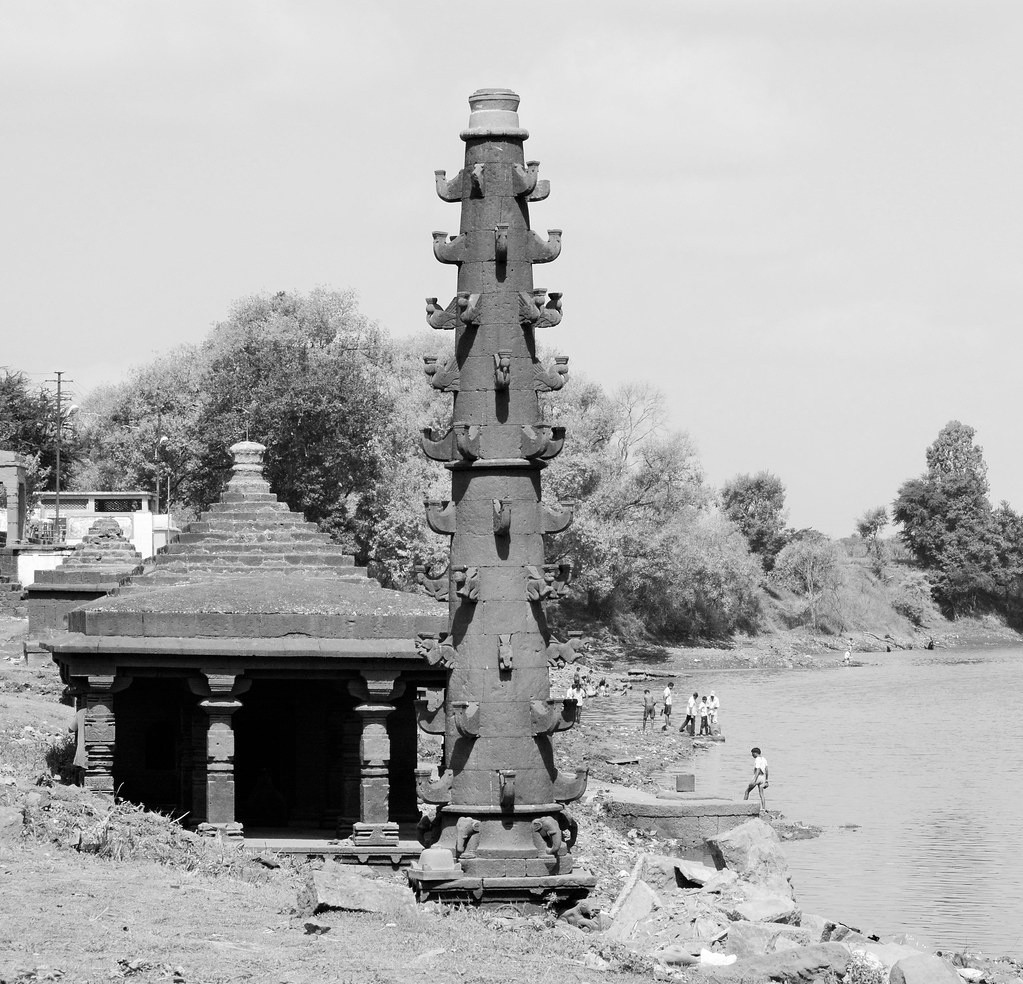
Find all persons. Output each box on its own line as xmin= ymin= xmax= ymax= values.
xmin=846 ymin=637 xmax=854 ymax=652
xmin=698 ymin=696 xmax=709 ymax=737
xmin=743 ymin=748 xmax=770 ymax=811
xmin=843 ymin=647 xmax=852 ymax=666
xmin=707 ymin=690 xmax=722 ymax=737
xmin=572 ymin=685 xmax=585 ymax=724
xmin=678 ymin=692 xmax=699 ymax=737
xmin=567 ymin=683 xmax=576 ymax=700
xmin=572 ymin=665 xmax=630 ymax=698
xmin=662 ymin=682 xmax=674 ymax=728
xmin=642 ymin=689 xmax=657 ymax=731
